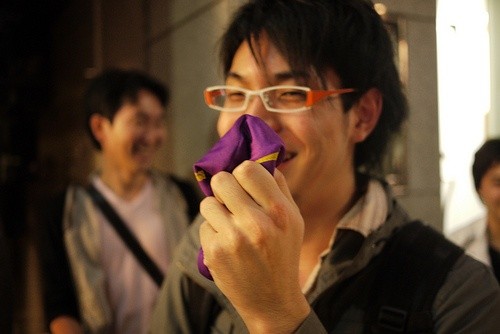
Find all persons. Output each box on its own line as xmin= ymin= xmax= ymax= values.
xmin=20 ymin=66 xmax=202 ymax=334
xmin=445 ymin=136 xmax=500 ymax=275
xmin=143 ymin=3 xmax=500 ymax=333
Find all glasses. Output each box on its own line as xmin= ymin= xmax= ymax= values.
xmin=204 ymin=84 xmax=355 ymax=112
